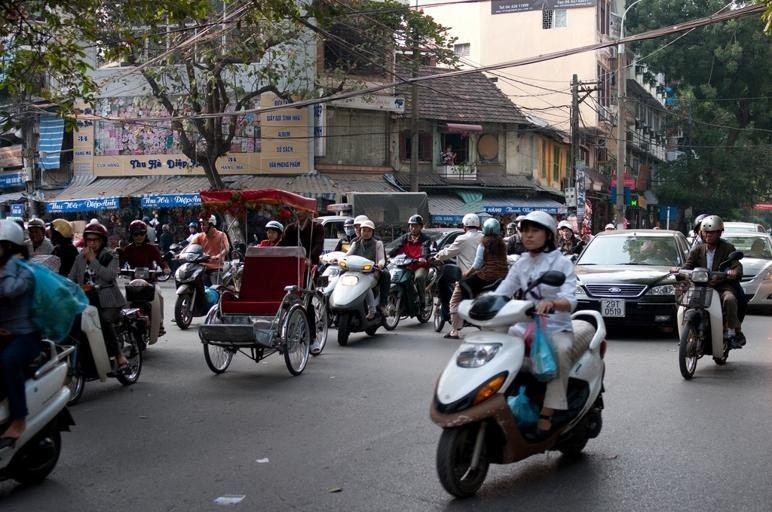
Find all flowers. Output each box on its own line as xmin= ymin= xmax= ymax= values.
xmin=440 ymin=146 xmax=457 ymax=163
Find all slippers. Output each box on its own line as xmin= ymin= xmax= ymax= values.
xmin=443 ymin=331 xmax=459 ymax=339
xmin=309 ymin=340 xmax=320 ymax=354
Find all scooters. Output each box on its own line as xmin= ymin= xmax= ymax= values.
xmin=318 ymin=215 xmax=590 ymax=333
xmin=668 ymin=249 xmax=741 ymax=382
xmin=427 ymin=265 xmax=608 ymax=498
xmin=333 ymin=258 xmax=390 ymax=346
xmin=171 ymin=244 xmax=245 ymax=331
xmin=65 ymin=280 xmax=153 ymax=407
xmin=1 ymin=338 xmax=78 ymax=487
xmin=117 ymin=265 xmax=173 ymax=351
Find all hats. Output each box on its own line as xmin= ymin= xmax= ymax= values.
xmin=605 ymin=223 xmax=615 ymax=230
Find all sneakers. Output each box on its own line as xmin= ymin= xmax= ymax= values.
xmin=728 ymin=331 xmax=747 ymax=349
xmin=535 ymin=414 xmax=554 ymax=439
xmin=365 ymin=301 xmax=427 ymax=321
xmin=0 ymin=418 xmax=28 ymax=448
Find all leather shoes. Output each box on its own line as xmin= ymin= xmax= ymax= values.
xmin=116 ymin=351 xmax=130 ymax=370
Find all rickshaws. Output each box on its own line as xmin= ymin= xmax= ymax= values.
xmin=196 ymin=187 xmax=331 ymax=377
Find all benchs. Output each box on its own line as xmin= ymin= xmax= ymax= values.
xmin=222 ymin=245 xmax=307 ymax=315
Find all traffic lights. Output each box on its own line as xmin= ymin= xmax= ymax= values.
xmin=630 ymin=195 xmax=639 ymax=208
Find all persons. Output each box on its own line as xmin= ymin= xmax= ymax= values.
xmin=253 ymin=221 xmax=283 ymax=247
xmin=274 ymin=212 xmax=324 ymax=355
xmin=493 ymin=211 xmax=576 ymax=447
xmin=743 ymin=239 xmax=771 ymax=257
xmin=178 ymin=213 xmax=229 ymax=296
xmin=320 ymin=213 xmax=526 ymax=338
xmin=653 ymin=241 xmax=670 ymax=262
xmin=678 ymin=213 xmax=746 ymax=346
xmin=1 ymin=217 xmax=174 ymax=446
xmin=557 ymin=221 xmax=583 ymax=262
xmin=671 ymin=215 xmax=743 ymax=349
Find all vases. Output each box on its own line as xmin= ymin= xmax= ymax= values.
xmin=448 ymin=161 xmax=455 ymax=166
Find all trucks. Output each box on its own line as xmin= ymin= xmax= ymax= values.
xmin=309 ymin=188 xmax=432 ymax=250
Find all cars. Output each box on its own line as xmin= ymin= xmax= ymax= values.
xmin=714 ymin=220 xmax=772 ymax=311
xmin=566 ymin=228 xmax=691 ymax=334
xmin=686 ymin=222 xmax=769 ymax=248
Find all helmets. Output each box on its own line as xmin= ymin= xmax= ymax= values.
xmin=462 ymin=213 xmax=481 ymax=228
xmin=188 ymin=222 xmax=199 ymax=229
xmin=557 ymin=219 xmax=573 ymax=233
xmin=521 ymin=211 xmax=558 ymax=242
xmin=0 ymin=219 xmax=26 ymax=246
xmin=82 ymin=222 xmax=108 ymax=242
xmin=506 ymin=215 xmax=526 ymax=231
xmin=700 ymin=215 xmax=725 ymax=232
xmin=27 ymin=218 xmax=46 ymax=234
xmin=693 ymin=214 xmax=708 ymax=233
xmin=265 ymin=220 xmax=284 ymax=234
xmin=128 ymin=220 xmax=147 ymax=237
xmin=198 ymin=214 xmax=217 ymax=226
xmin=343 ymin=215 xmax=375 ymax=230
xmin=481 ymin=218 xmax=501 ymax=236
xmin=50 ymin=218 xmax=73 ymax=238
xmin=407 ymin=214 xmax=424 ymax=226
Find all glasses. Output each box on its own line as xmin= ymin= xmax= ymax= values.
xmin=133 ymin=230 xmax=146 ymax=237
